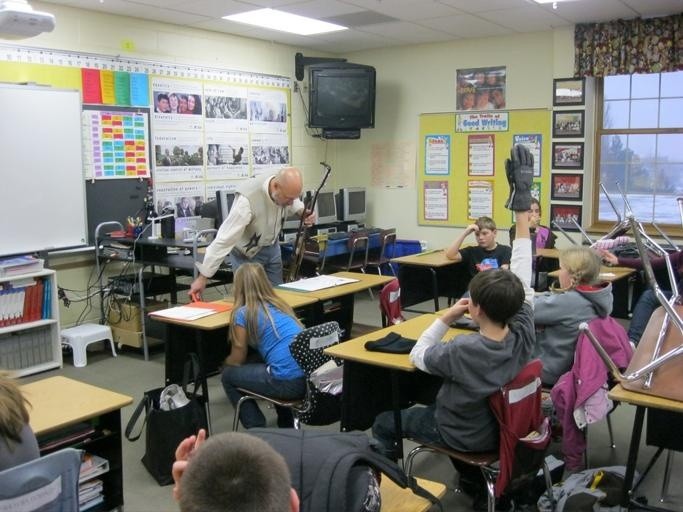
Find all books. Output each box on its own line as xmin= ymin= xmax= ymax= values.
xmin=275 ymin=274 xmax=361 ymax=294
xmin=79 ymin=455 xmax=111 ymax=512
xmin=0 ymin=255 xmax=55 ymax=372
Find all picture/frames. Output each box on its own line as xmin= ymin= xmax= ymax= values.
xmin=552 ymin=110 xmax=586 ymax=140
xmin=550 ymin=173 xmax=584 ymax=202
xmin=552 ymin=76 xmax=586 ymax=107
xmin=549 ymin=204 xmax=584 ymax=234
xmin=552 ymin=142 xmax=586 ymax=171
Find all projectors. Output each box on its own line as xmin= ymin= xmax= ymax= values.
xmin=0 ymin=0 xmax=55 ymax=40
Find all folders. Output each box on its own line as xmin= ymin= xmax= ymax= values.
xmin=79 ymin=454 xmax=111 ymax=484
xmin=78 ymin=478 xmax=104 ymax=512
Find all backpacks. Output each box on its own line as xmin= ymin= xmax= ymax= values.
xmin=248 ymin=427 xmax=442 ymax=512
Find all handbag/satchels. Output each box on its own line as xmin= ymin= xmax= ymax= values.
xmin=125 ymin=353 xmax=209 ymax=487
xmin=514 ymin=421 xmax=552 ymax=473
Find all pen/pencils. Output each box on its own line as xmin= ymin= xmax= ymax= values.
xmin=127 ymin=217 xmax=144 ymax=230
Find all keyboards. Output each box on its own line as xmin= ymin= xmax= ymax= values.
xmin=327 ymin=232 xmax=347 ymax=240
xmin=305 ymin=241 xmax=319 ymax=252
xmin=361 ymin=228 xmax=383 ymax=234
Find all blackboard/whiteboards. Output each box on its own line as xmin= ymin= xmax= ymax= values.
xmin=81 ymin=104 xmax=154 ymax=246
xmin=0 ymin=82 xmax=90 ymax=258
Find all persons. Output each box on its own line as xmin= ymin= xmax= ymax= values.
xmin=593 ymin=247 xmax=683 ymax=351
xmin=159 ymin=199 xmax=204 ymax=217
xmin=456 ymin=68 xmax=505 ymax=110
xmin=186 ymin=164 xmax=317 ymax=301
xmin=446 ymin=216 xmax=510 ymax=280
xmin=154 ymin=142 xmax=291 ymax=167
xmin=370 ymin=142 xmax=539 ymax=467
xmin=219 ymin=260 xmax=310 ymax=429
xmin=166 ymin=425 xmax=305 ymax=512
xmin=0 ymin=366 xmax=44 ymax=474
xmin=550 ymin=86 xmax=583 ymax=232
xmin=508 ymin=198 xmax=558 ymax=287
xmin=155 ymin=91 xmax=288 ymax=125
xmin=531 ymin=244 xmax=616 ymax=392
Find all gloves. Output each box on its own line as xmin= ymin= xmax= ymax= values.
xmin=505 ymin=144 xmax=534 ymax=210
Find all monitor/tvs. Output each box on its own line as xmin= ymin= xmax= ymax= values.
xmin=308 ymin=63 xmax=376 ymax=129
xmin=335 ymin=187 xmax=367 ymax=221
xmin=314 ymin=190 xmax=337 ymax=224
xmin=200 ymin=189 xmax=235 ymax=229
xmin=282 ymin=194 xmax=303 ymax=229
